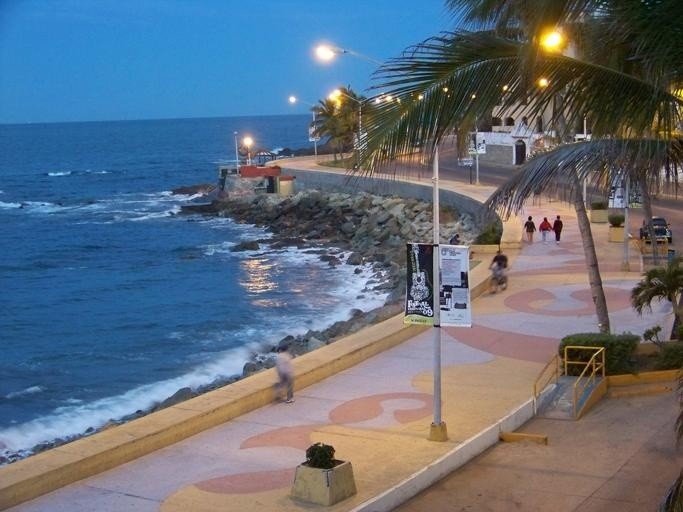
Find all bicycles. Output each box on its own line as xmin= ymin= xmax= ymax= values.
xmin=489 ymin=268 xmax=508 ymax=294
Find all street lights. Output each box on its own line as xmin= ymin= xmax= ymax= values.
xmin=333 ymin=89 xmax=362 ymax=175
xmin=240 ymin=134 xmax=254 ymax=166
xmin=311 ymin=43 xmax=447 ymax=443
xmin=289 ymin=96 xmax=317 ymax=156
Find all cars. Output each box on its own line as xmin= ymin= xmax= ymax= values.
xmin=639 ymin=216 xmax=672 ymax=244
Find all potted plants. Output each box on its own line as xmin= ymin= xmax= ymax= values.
xmin=608 ymin=214 xmax=625 ymax=241
xmin=290 ymin=443 xmax=356 ymax=505
xmin=590 ymin=202 xmax=609 ymax=222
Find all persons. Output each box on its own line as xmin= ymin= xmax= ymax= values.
xmin=538 ymin=216 xmax=552 ymax=243
xmin=522 ymin=215 xmax=535 ymax=245
xmin=270 ymin=342 xmax=294 ymax=405
xmin=487 ymin=250 xmax=507 ymax=287
xmin=448 ymin=235 xmax=460 ymax=246
xmin=551 ymin=215 xmax=562 ymax=244
